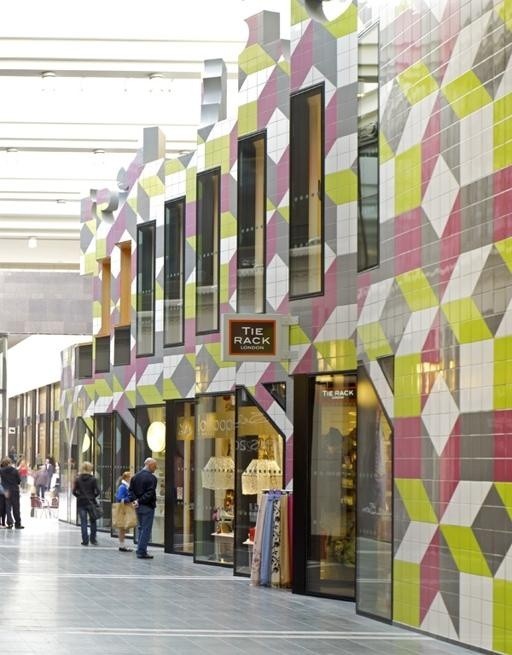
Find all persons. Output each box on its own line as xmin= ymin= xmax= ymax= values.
xmin=72 ymin=461 xmax=100 ymax=546
xmin=0 ymin=446 xmax=53 ymax=529
xmin=115 ymin=471 xmax=134 ymax=552
xmin=128 ymin=457 xmax=158 ymax=559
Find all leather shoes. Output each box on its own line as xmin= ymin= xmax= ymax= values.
xmin=119 ymin=547 xmax=133 ymax=552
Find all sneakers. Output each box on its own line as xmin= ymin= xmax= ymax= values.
xmin=81 ymin=540 xmax=99 ymax=546
xmin=0 ymin=523 xmax=24 ymax=529
xmin=137 ymin=554 xmax=153 ymax=559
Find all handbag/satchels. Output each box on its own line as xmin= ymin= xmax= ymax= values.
xmin=89 ymin=504 xmax=104 ymax=520
xmin=111 ymin=499 xmax=138 ymax=528
